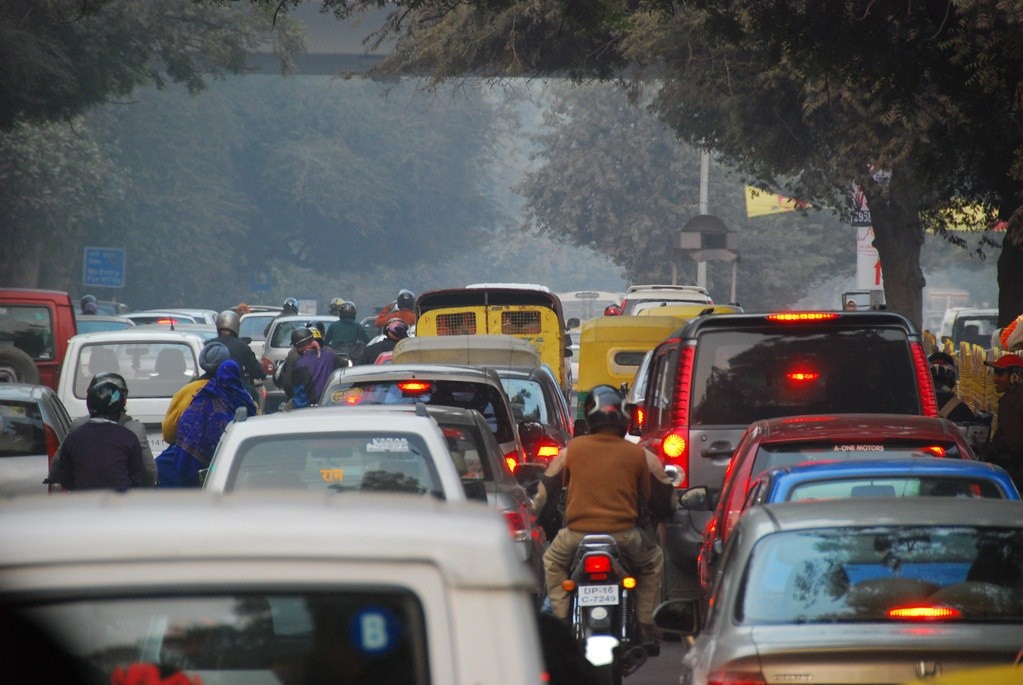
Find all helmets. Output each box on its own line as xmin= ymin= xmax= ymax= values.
xmin=284 ymin=297 xmax=298 ymax=313
xmin=585 ymin=385 xmax=623 ymax=410
xmin=307 ymin=320 xmax=325 ymax=335
xmin=604 ymin=305 xmax=622 ymax=316
xmin=80 ymin=295 xmax=95 ymax=309
xmin=339 ymin=301 xmax=357 ymax=320
xmin=329 ymin=298 xmax=344 ymax=315
xmin=87 ymin=382 xmax=126 ymax=415
xmin=217 ymin=310 xmax=240 ymax=336
xmin=308 ymin=327 xmax=322 ymax=340
xmin=927 ymin=352 xmax=957 ymax=389
xmin=384 ymin=318 xmax=408 ymax=335
xmin=398 ymin=292 xmax=415 ymax=310
xmin=88 ymin=373 xmax=128 ymax=395
xmin=587 ymin=392 xmax=629 ymax=438
xmin=291 ymin=327 xmax=313 ymax=347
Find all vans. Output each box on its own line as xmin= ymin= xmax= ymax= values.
xmin=0 ymin=491 xmax=548 ymax=685
xmin=56 ymin=328 xmax=205 ymax=457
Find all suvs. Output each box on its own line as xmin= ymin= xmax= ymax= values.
xmin=630 ymin=308 xmax=937 ymax=624
xmin=0 ymin=287 xmax=78 ymax=414
xmin=199 ymin=402 xmax=465 ymax=506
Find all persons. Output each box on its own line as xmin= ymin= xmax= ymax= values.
xmin=846 ymin=299 xmax=1023 ymax=497
xmin=528 ymin=383 xmax=677 ymax=654
xmin=44 ymin=289 xmax=419 ymax=494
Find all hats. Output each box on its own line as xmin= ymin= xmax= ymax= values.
xmin=983 ymin=354 xmax=1023 ymax=374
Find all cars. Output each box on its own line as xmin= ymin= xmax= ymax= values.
xmin=364 ymin=317 xmax=412 ymax=403
xmin=651 ymin=495 xmax=1023 ymax=685
xmin=705 ymin=459 xmax=1023 ymax=588
xmin=315 ymin=366 xmax=526 ymax=473
xmin=72 ymin=299 xmax=341 ymax=408
xmin=626 ymin=353 xmax=653 ymax=403
xmin=0 ymin=380 xmax=73 ymax=496
xmin=494 ymin=367 xmax=574 ymax=494
xmin=679 ymin=412 xmax=986 ymax=622
xmin=940 ymin=306 xmax=998 ymax=361
xmin=307 ymin=409 xmax=547 ymax=613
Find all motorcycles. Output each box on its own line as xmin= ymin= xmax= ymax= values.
xmin=562 ymin=537 xmax=649 ymax=685
xmin=415 ymin=289 xmax=737 ymax=418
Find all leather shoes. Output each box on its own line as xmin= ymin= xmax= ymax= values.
xmin=638 ymin=625 xmax=662 ymax=646
